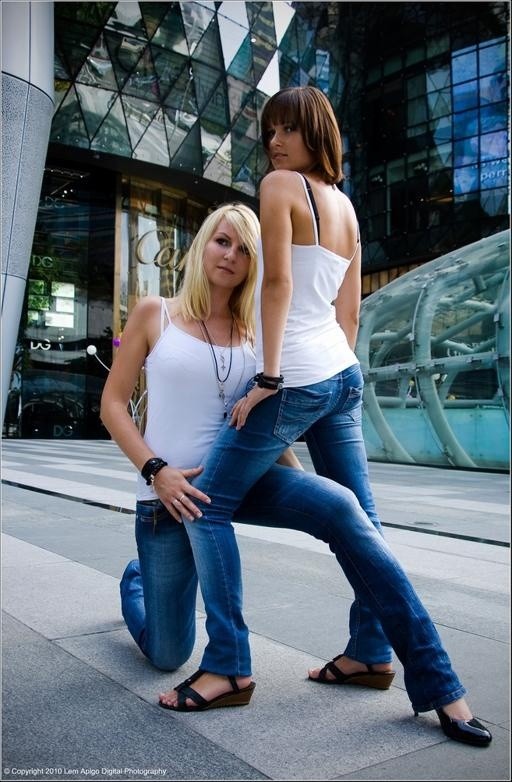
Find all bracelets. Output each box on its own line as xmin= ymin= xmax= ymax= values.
xmin=139 ymin=457 xmax=162 ymax=480
xmin=145 ymin=461 xmax=168 ymax=486
xmin=256 ymin=380 xmax=278 ymax=391
xmin=257 ymin=372 xmax=285 ymax=383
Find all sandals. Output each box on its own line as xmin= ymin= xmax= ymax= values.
xmin=159 ymin=668 xmax=256 ymax=712
xmin=308 ymin=653 xmax=395 ymax=689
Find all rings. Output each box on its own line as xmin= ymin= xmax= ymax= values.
xmin=172 ymin=499 xmax=178 ymax=506
xmin=179 ymin=493 xmax=184 ymax=502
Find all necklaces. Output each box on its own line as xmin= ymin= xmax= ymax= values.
xmin=198 ymin=311 xmax=244 ymax=420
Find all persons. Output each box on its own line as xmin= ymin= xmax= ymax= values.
xmin=158 ymin=87 xmax=395 ymax=713
xmin=97 ymin=204 xmax=494 ymax=749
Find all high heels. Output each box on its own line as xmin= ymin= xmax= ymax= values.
xmin=413 ymin=695 xmax=493 ymax=749
